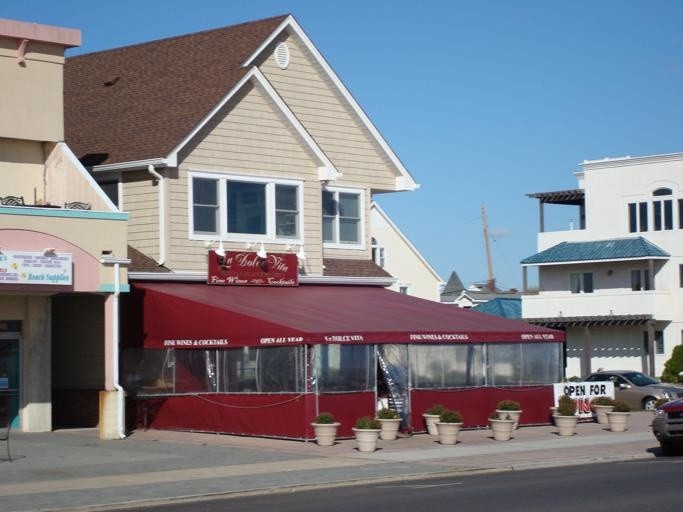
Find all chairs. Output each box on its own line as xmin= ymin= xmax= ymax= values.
xmin=0 ymin=195 xmax=91 ymax=210
xmin=0 ymin=417 xmax=13 ymax=462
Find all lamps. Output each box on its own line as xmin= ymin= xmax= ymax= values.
xmin=205 ymin=237 xmax=306 ymax=260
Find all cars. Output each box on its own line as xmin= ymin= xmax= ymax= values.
xmin=652 ymin=397 xmax=682 ymax=449
xmin=578 ymin=367 xmax=681 ymax=413
